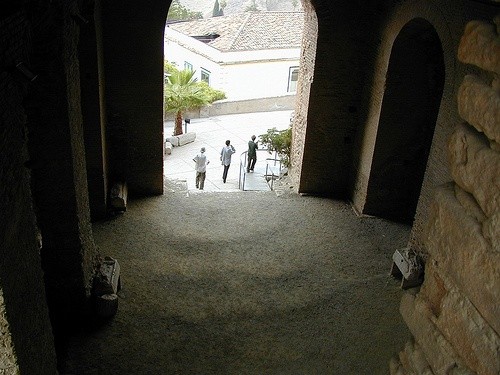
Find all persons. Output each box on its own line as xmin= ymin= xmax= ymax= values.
xmin=246 ymin=135 xmax=258 ymax=173
xmin=221 ymin=140 xmax=236 ymax=184
xmin=193 ymin=147 xmax=210 ymax=190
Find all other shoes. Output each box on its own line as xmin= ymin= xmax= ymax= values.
xmin=251 ymin=168 xmax=253 ymax=171
xmin=197 ymin=186 xmax=198 ymax=188
xmin=248 ymin=171 xmax=250 ymax=172
xmin=224 ymin=180 xmax=226 ymax=182
xmin=199 ymin=188 xmax=202 ymax=189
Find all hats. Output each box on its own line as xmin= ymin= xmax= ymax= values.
xmin=200 ymin=147 xmax=206 ymax=152
xmin=251 ymin=135 xmax=256 ymax=138
